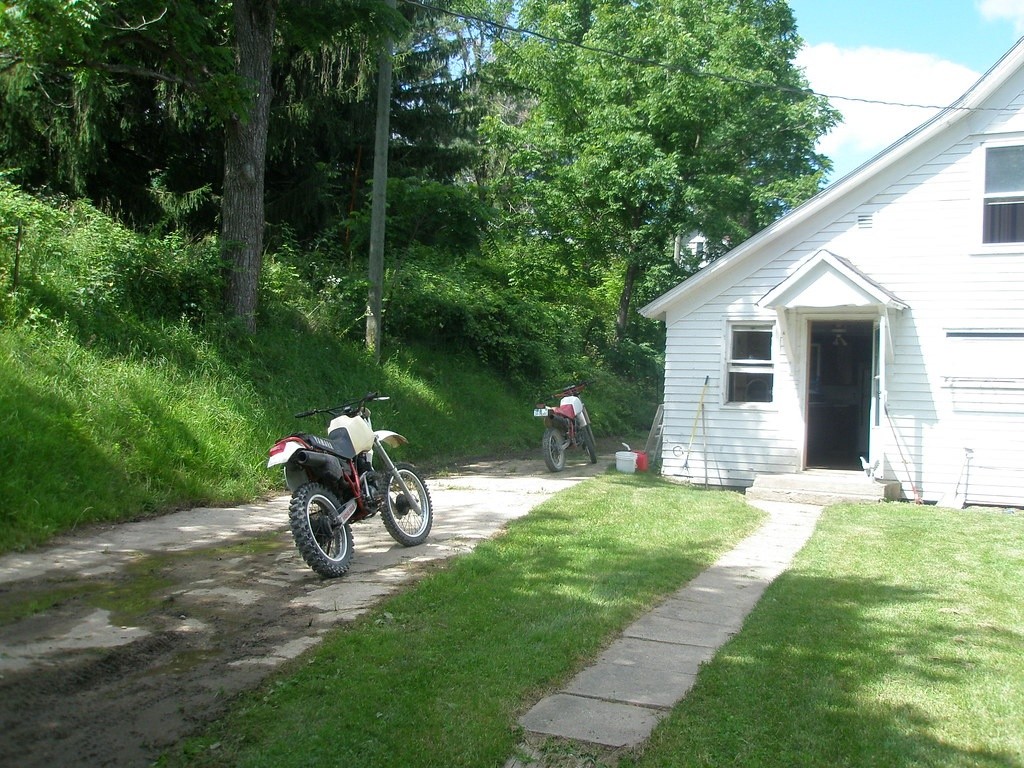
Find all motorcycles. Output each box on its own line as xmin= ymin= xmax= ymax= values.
xmin=533 ymin=380 xmax=598 ymax=472
xmin=266 ymin=392 xmax=434 ymax=579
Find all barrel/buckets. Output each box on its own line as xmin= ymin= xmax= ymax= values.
xmin=615 ymin=451 xmax=638 ymax=474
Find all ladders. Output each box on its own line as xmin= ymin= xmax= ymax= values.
xmin=644 ymin=404 xmax=664 ymax=462
